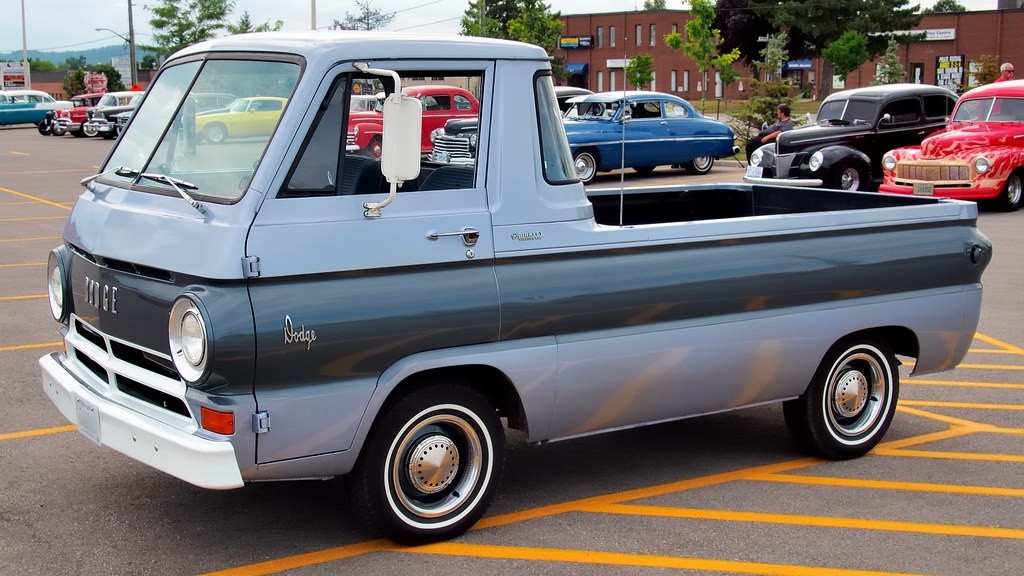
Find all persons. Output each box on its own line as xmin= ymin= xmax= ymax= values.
xmin=745 ymin=103 xmax=793 ymax=172
xmin=991 ymin=63 xmax=1014 ymax=114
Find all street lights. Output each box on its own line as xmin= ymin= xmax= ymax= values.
xmin=95 ymin=27 xmax=138 ymax=89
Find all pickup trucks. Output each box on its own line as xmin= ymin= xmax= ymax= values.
xmin=36 ymin=26 xmax=995 ymax=545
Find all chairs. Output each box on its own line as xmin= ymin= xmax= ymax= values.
xmin=344 ymin=155 xmax=473 ymax=195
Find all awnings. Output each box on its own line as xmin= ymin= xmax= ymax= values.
xmin=783 ymin=59 xmax=813 ymax=70
xmin=563 ymin=63 xmax=588 ymax=74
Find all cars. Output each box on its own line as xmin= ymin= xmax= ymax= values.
xmin=180 ymin=96 xmax=290 ymax=145
xmin=37 ymin=89 xmax=149 ymax=140
xmin=344 ymin=82 xmax=480 ymax=162
xmin=878 ymin=78 xmax=1024 ymax=211
xmin=743 ymin=81 xmax=976 ymax=191
xmin=182 ymin=91 xmax=239 ymax=117
xmin=561 ymin=89 xmax=741 ymax=186
xmin=0 ymin=89 xmax=81 ymax=127
xmin=425 ymin=84 xmax=611 ymax=169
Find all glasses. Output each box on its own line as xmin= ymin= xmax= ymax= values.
xmin=1003 ymin=70 xmax=1015 ymax=73
